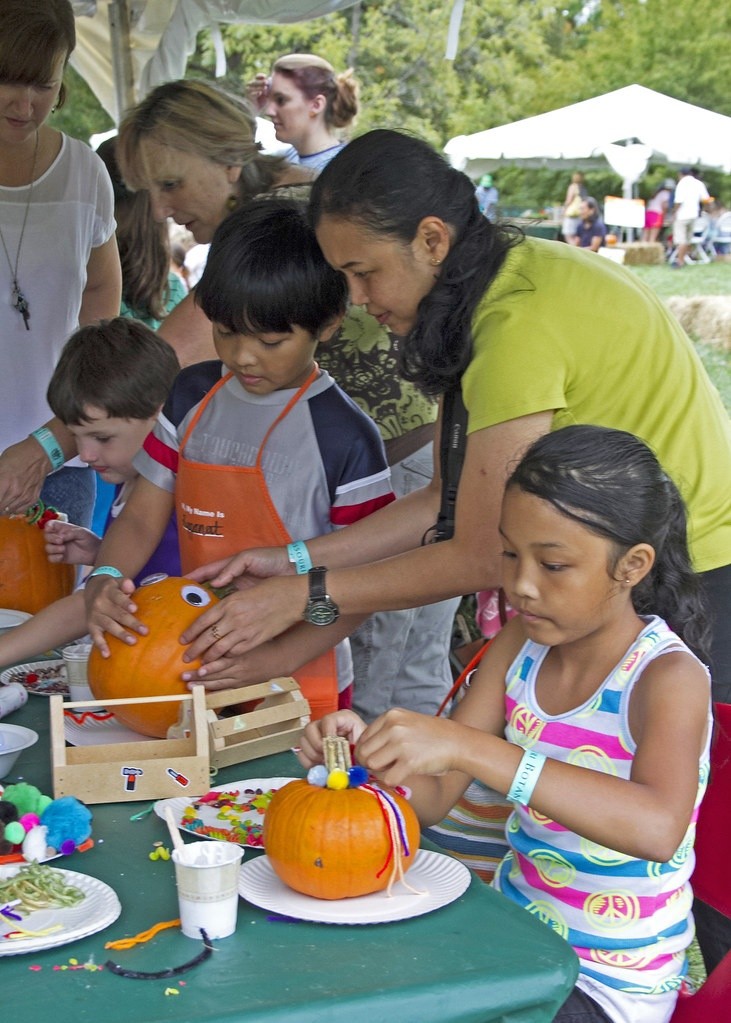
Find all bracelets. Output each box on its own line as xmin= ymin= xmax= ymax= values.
xmin=30 ymin=428 xmax=65 ymax=468
xmin=73 ymin=582 xmax=86 ymax=592
xmin=286 ymin=540 xmax=313 ymax=575
xmin=85 ymin=566 xmax=123 ymax=582
xmin=505 ymin=749 xmax=547 ymax=807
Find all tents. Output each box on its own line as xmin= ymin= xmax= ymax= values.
xmin=443 ymin=83 xmax=731 ymax=243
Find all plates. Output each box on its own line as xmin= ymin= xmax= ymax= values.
xmin=0 ymin=861 xmax=123 ymax=960
xmin=0 ymin=659 xmax=71 ymax=698
xmin=153 ymin=776 xmax=307 ymax=850
xmin=237 ymin=848 xmax=471 ymax=925
xmin=0 ymin=722 xmax=40 ymax=780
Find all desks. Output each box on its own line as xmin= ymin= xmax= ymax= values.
xmin=0 ymin=609 xmax=579 ymax=1023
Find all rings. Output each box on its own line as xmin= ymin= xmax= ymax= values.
xmin=211 ymin=626 xmax=222 ymax=640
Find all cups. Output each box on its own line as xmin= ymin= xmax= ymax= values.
xmin=171 ymin=839 xmax=245 ymax=940
xmin=62 ymin=643 xmax=107 ymax=713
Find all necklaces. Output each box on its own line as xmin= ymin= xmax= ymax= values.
xmin=0 ymin=129 xmax=42 ymax=333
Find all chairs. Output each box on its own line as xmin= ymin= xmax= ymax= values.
xmin=666 ymin=212 xmax=731 ymax=265
xmin=669 ymin=702 xmax=731 ymax=1023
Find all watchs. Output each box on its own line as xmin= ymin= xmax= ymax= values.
xmin=302 ymin=566 xmax=339 ymax=625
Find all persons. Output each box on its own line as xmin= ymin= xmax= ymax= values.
xmin=244 ymin=53 xmax=358 ymax=174
xmin=77 ymin=192 xmax=398 ymax=724
xmin=0 ymin=78 xmax=464 ymax=727
xmin=0 ymin=0 xmax=125 ymax=593
xmin=0 ymin=319 xmax=185 ymax=671
xmin=560 ymin=171 xmax=607 ymax=253
xmin=475 ymin=174 xmax=498 ymax=224
xmin=294 ymin=424 xmax=714 ymax=1023
xmin=93 ymin=136 xmax=210 ymax=335
xmin=173 ymin=127 xmax=731 ymax=704
xmin=641 ymin=164 xmax=731 ymax=270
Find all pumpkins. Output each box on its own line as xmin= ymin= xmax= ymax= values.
xmin=0 ymin=512 xmax=76 ymax=613
xmin=605 ymin=233 xmax=616 ymax=245
xmin=264 ymin=735 xmax=420 ymax=901
xmin=86 ymin=573 xmax=238 ymax=739
